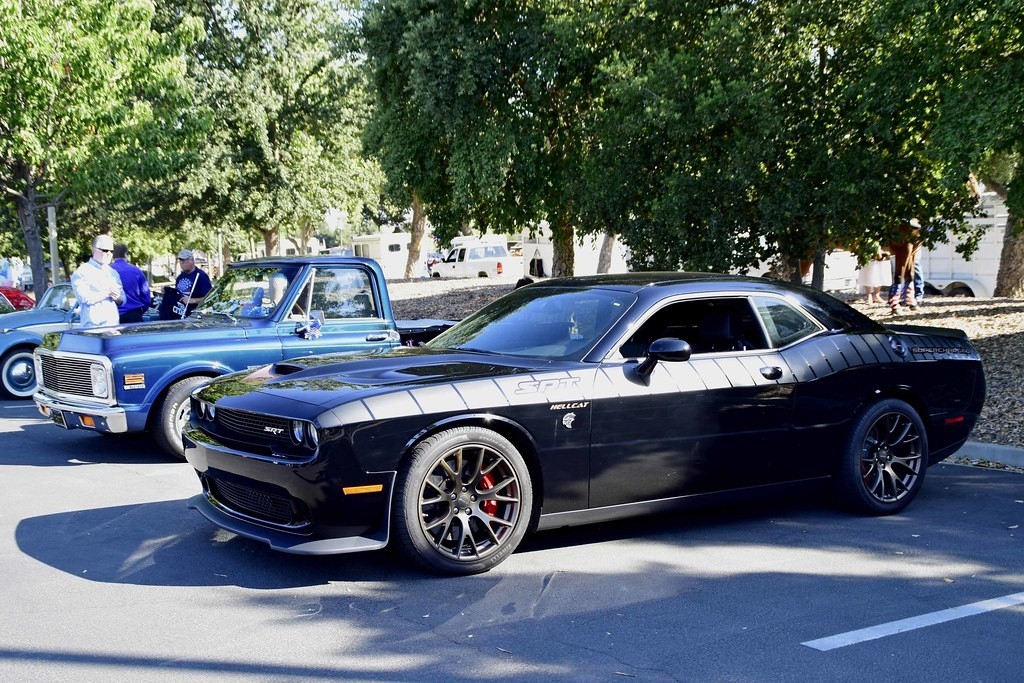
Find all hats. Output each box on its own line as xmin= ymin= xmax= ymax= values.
xmin=910 ymin=218 xmax=921 ymax=228
xmin=176 ymin=250 xmax=193 ymax=260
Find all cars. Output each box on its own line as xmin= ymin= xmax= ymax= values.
xmin=0 ymin=286 xmax=35 ymax=315
xmin=182 ymin=271 xmax=987 ymax=575
xmin=0 ymin=284 xmax=162 ymax=398
xmin=313 ymin=248 xmax=354 ymax=277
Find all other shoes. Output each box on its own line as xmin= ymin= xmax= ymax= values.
xmin=873 ymin=297 xmax=884 ymax=303
xmin=865 ymin=295 xmax=874 ymax=305
xmin=909 ymin=305 xmax=920 ymax=310
xmin=891 ymin=308 xmax=901 ymax=315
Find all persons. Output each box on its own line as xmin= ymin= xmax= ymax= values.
xmin=71 ymin=234 xmax=153 ymax=327
xmin=857 ymin=223 xmax=928 ymax=315
xmin=175 ymin=249 xmax=213 ymax=318
xmin=269 ymin=267 xmax=309 ymax=315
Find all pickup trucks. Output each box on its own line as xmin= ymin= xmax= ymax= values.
xmin=430 ymin=243 xmax=523 ymax=278
xmin=31 ymin=255 xmax=577 ymax=461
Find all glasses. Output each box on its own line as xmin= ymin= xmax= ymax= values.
xmin=911 ymin=227 xmax=919 ymax=230
xmin=93 ymin=246 xmax=115 ymax=254
